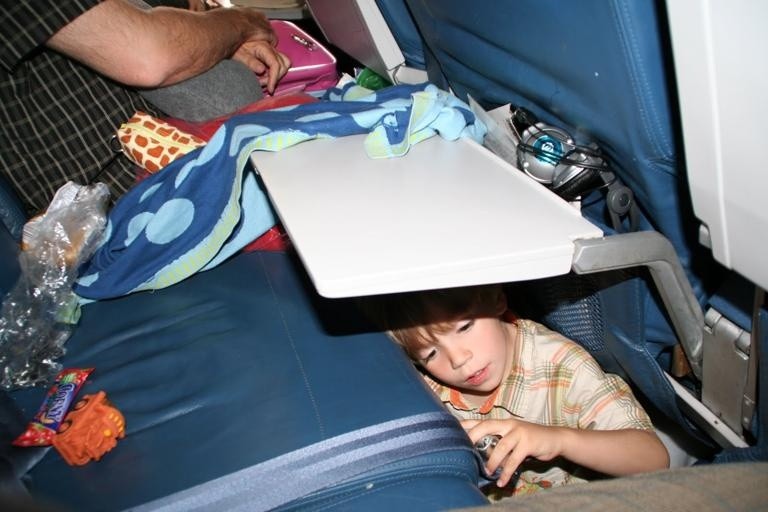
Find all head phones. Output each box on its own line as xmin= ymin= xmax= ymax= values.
xmin=510 ymin=107 xmax=609 ymax=206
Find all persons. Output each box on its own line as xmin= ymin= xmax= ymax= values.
xmin=376 ymin=283 xmax=671 ymax=504
xmin=0 ymin=0 xmax=289 ymax=225
